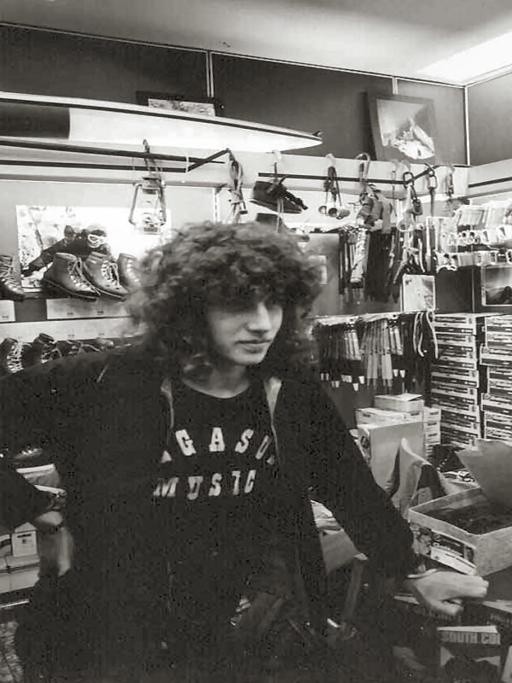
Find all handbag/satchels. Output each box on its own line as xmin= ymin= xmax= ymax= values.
xmin=314 ymin=556 xmax=441 ymax=682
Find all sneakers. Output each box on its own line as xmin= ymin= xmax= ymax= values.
xmin=249 ymin=182 xmax=301 ymax=214
xmin=1 ymin=227 xmax=142 ymax=302
xmin=0 ymin=334 xmax=113 ymax=377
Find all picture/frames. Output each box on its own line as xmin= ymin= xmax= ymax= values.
xmin=366 ymin=90 xmax=444 ymax=165
xmin=136 ymin=90 xmax=224 ymax=118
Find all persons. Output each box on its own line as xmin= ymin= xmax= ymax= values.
xmin=0 ymin=221 xmax=489 ymax=683
